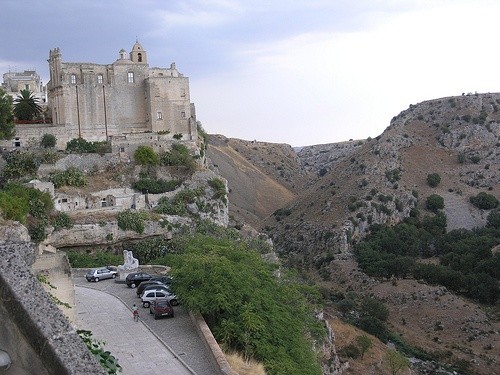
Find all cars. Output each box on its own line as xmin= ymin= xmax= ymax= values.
xmin=140 ymin=285 xmax=171 ymax=297
xmin=126 ymin=272 xmax=152 ymax=288
xmin=149 ymin=276 xmax=173 ymax=285
xmin=143 ymin=290 xmax=179 ymax=308
xmin=149 ymin=299 xmax=174 ymax=320
xmin=85 ymin=268 xmax=119 ymax=282
xmin=136 ymin=281 xmax=164 ymax=294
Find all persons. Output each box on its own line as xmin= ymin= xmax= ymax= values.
xmin=132 ymin=304 xmax=139 ymax=322
